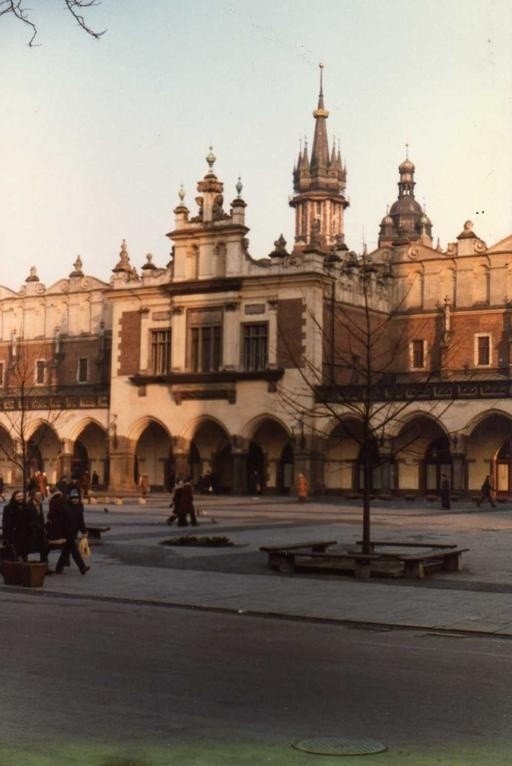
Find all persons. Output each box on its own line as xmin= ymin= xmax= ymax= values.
xmin=45 ymin=486 xmax=66 ymax=540
xmin=25 ymin=487 xmax=56 ymax=575
xmin=476 ymin=475 xmax=496 ymax=508
xmin=3 ymin=490 xmax=30 ymax=563
xmin=178 ymin=477 xmax=198 ymax=526
xmin=167 ymin=478 xmax=184 ymax=527
xmin=92 ymin=470 xmax=99 ymax=485
xmin=69 ymin=478 xmax=81 ymax=493
xmin=203 ymin=470 xmax=212 ymax=495
xmin=141 ymin=473 xmax=152 ymax=494
xmin=296 ymin=473 xmax=309 ymax=505
xmin=440 ymin=474 xmax=450 ymax=510
xmin=41 ymin=472 xmax=48 ymax=499
xmin=57 ymin=489 xmax=91 ymax=576
xmin=28 ymin=470 xmax=40 ymax=491
xmin=253 ymin=471 xmax=261 ymax=496
xmin=57 ymin=475 xmax=70 ymax=498
xmin=83 ymin=469 xmax=92 ymax=495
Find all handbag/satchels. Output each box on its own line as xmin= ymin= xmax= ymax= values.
xmin=0 ymin=543 xmax=16 ymax=561
xmin=79 ymin=538 xmax=91 ymax=558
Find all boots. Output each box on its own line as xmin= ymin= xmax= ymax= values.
xmin=78 ymin=560 xmax=90 ymax=575
xmin=56 ymin=557 xmax=65 ymax=572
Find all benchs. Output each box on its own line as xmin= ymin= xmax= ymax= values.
xmin=259 ymin=539 xmax=470 ymax=580
xmin=0 ymin=521 xmax=111 ymax=560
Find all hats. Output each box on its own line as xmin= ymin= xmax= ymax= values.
xmin=70 ymin=488 xmax=80 ymax=498
